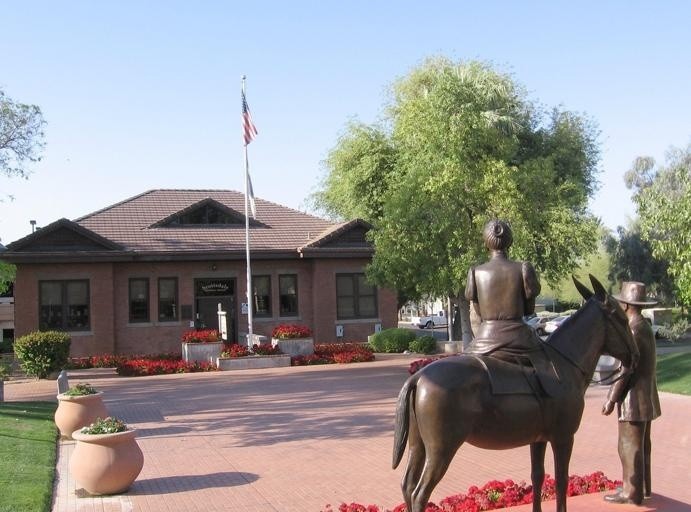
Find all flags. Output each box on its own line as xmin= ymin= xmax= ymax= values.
xmin=239 ymin=77 xmax=259 ymax=148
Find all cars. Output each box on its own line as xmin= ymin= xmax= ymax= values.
xmin=524 ymin=309 xmax=576 ymax=336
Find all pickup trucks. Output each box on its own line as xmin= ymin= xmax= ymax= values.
xmin=412 ymin=307 xmax=458 ymax=329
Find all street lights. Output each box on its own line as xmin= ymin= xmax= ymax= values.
xmin=29 ymin=220 xmax=36 ymax=234
xmin=35 ymin=227 xmax=39 ymax=230
xmin=427 ymin=296 xmax=435 ymax=339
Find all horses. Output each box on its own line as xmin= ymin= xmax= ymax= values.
xmin=390 ymin=273 xmax=641 ymax=511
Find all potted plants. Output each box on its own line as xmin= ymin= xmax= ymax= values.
xmin=54 ymin=385 xmax=109 ymax=437
xmin=72 ymin=418 xmax=144 ymax=496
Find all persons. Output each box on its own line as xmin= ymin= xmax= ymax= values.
xmin=600 ymin=280 xmax=663 ymax=507
xmin=463 ymin=218 xmax=547 ymax=357
